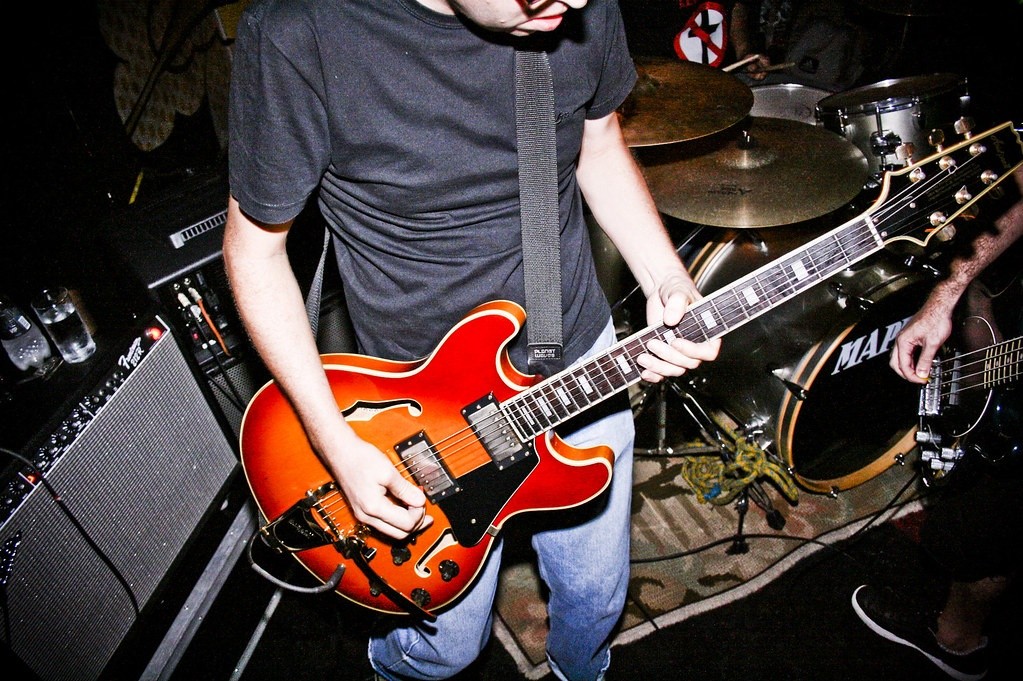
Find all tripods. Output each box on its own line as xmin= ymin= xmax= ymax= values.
xmin=634 ymin=379 xmax=786 ymax=530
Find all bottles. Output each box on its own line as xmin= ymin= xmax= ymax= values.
xmin=0 ymin=293 xmax=51 ymax=371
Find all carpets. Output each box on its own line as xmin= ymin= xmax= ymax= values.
xmin=491 ymin=222 xmax=929 ymax=680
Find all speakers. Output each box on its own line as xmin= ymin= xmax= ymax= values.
xmin=195 ymin=285 xmax=364 ymax=447
xmin=0 ymin=313 xmax=248 ymax=681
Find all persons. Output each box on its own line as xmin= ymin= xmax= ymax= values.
xmin=848 ymin=191 xmax=1023 ymax=681
xmin=221 ymin=0 xmax=720 ymax=681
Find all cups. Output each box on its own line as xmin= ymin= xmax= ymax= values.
xmin=31 ymin=287 xmax=95 ymax=363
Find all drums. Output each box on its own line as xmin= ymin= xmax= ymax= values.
xmin=675 ymin=226 xmax=959 ymax=500
xmin=813 ymin=70 xmax=976 ymax=198
xmin=744 ymin=82 xmax=838 ymax=130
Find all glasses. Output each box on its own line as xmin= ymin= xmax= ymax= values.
xmin=516 ymin=0 xmax=554 ymax=18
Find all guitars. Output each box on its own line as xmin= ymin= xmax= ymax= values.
xmin=912 ymin=309 xmax=1023 ymax=439
xmin=229 ymin=113 xmax=1023 ymax=628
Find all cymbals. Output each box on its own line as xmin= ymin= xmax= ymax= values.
xmin=616 ymin=52 xmax=757 ymax=153
xmin=632 ymin=114 xmax=873 ymax=231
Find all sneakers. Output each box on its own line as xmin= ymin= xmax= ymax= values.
xmin=853 ymin=584 xmax=989 ymax=681
